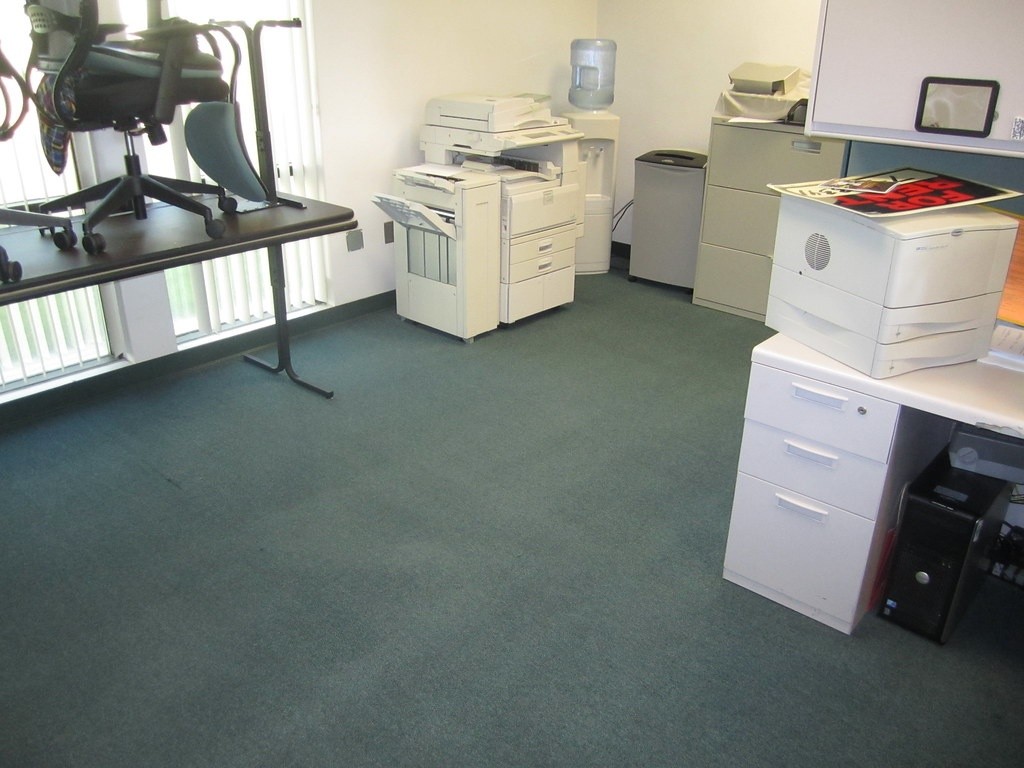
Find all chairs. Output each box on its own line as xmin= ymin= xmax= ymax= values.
xmin=67 ymin=0 xmax=270 ymax=208
xmin=0 ymin=46 xmax=78 ymax=286
xmin=22 ymin=0 xmax=245 ymax=252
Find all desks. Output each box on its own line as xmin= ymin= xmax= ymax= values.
xmin=0 ymin=185 xmax=358 ymax=399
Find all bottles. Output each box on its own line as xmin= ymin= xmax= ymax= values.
xmin=568 ymin=38 xmax=617 ymax=110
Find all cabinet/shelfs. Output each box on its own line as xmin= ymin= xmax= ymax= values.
xmin=720 ymin=327 xmax=1024 ymax=637
